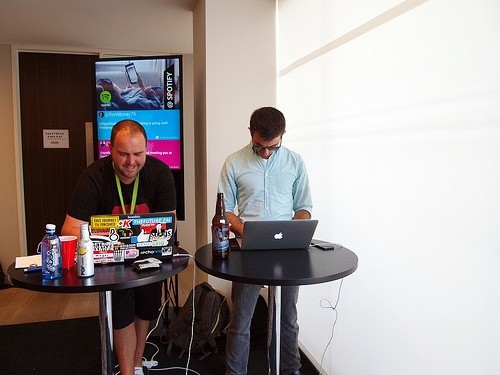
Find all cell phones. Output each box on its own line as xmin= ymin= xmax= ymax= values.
xmin=126 ymin=63 xmax=138 ymax=84
xmin=314 ymin=243 xmax=334 ymax=250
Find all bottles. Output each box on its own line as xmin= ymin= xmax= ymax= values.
xmin=41 ymin=224 xmax=62 ymax=280
xmin=212 ymin=193 xmax=230 ymax=260
xmin=77 ymin=223 xmax=94 ymax=277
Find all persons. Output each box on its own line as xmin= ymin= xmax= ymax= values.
xmin=62 ymin=119 xmax=177 ymax=375
xmin=151 ymin=223 xmax=165 ymax=236
xmin=217 ymin=108 xmax=313 ymax=375
xmin=96 ymin=72 xmax=163 ymax=110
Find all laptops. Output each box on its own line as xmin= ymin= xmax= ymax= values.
xmin=90 ymin=213 xmax=176 ymax=266
xmin=235 ymin=221 xmax=317 ymax=249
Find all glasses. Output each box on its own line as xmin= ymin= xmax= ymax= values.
xmin=254 ymin=133 xmax=283 ymax=150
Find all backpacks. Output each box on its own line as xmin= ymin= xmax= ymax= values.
xmin=159 ymin=281 xmax=230 ymax=362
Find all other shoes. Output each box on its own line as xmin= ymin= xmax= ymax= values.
xmin=135 ymin=366 xmax=144 ymax=374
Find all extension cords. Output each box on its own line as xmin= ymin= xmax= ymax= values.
xmin=142 ymin=360 xmax=159 ymax=366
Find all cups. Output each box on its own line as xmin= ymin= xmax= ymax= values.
xmin=57 ymin=236 xmax=76 ymax=270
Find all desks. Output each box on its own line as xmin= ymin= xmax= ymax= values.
xmin=9 ymin=247 xmax=190 ymax=375
xmin=193 ymin=237 xmax=360 ymax=375
